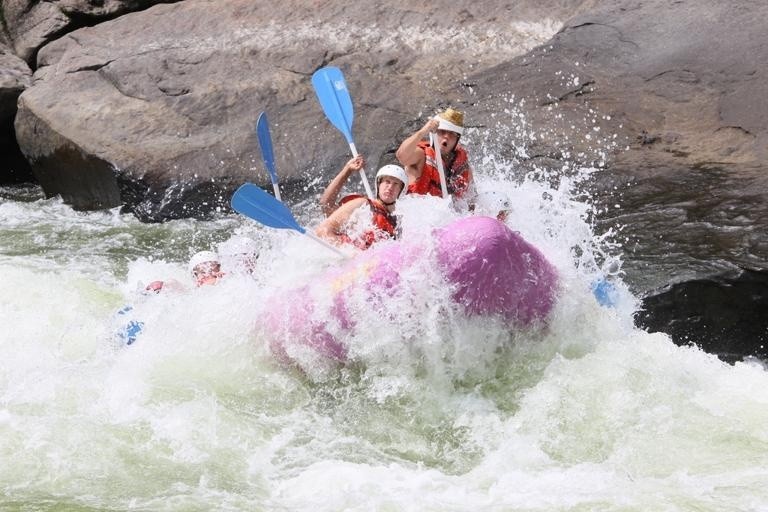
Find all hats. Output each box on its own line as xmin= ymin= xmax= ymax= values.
xmin=434 ymin=107 xmax=464 ymax=135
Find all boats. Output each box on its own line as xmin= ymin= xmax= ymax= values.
xmin=256 ymin=206 xmax=572 ymax=381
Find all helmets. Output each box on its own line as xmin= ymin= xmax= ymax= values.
xmin=190 ymin=237 xmax=259 ymax=269
xmin=146 ymin=281 xmax=163 ymax=290
xmin=374 ymin=165 xmax=407 ymax=189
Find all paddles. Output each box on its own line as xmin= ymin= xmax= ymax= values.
xmin=312 ymin=66 xmax=375 ymax=199
xmin=231 ymin=183 xmax=346 ymax=257
xmin=256 ymin=110 xmax=282 ymax=203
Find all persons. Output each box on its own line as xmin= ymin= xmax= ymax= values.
xmin=313 ymin=151 xmax=410 ymax=251
xmin=145 ymin=236 xmax=260 ymax=299
xmin=395 ymin=108 xmax=477 ymax=202
xmin=467 ymin=190 xmax=513 ymax=221
